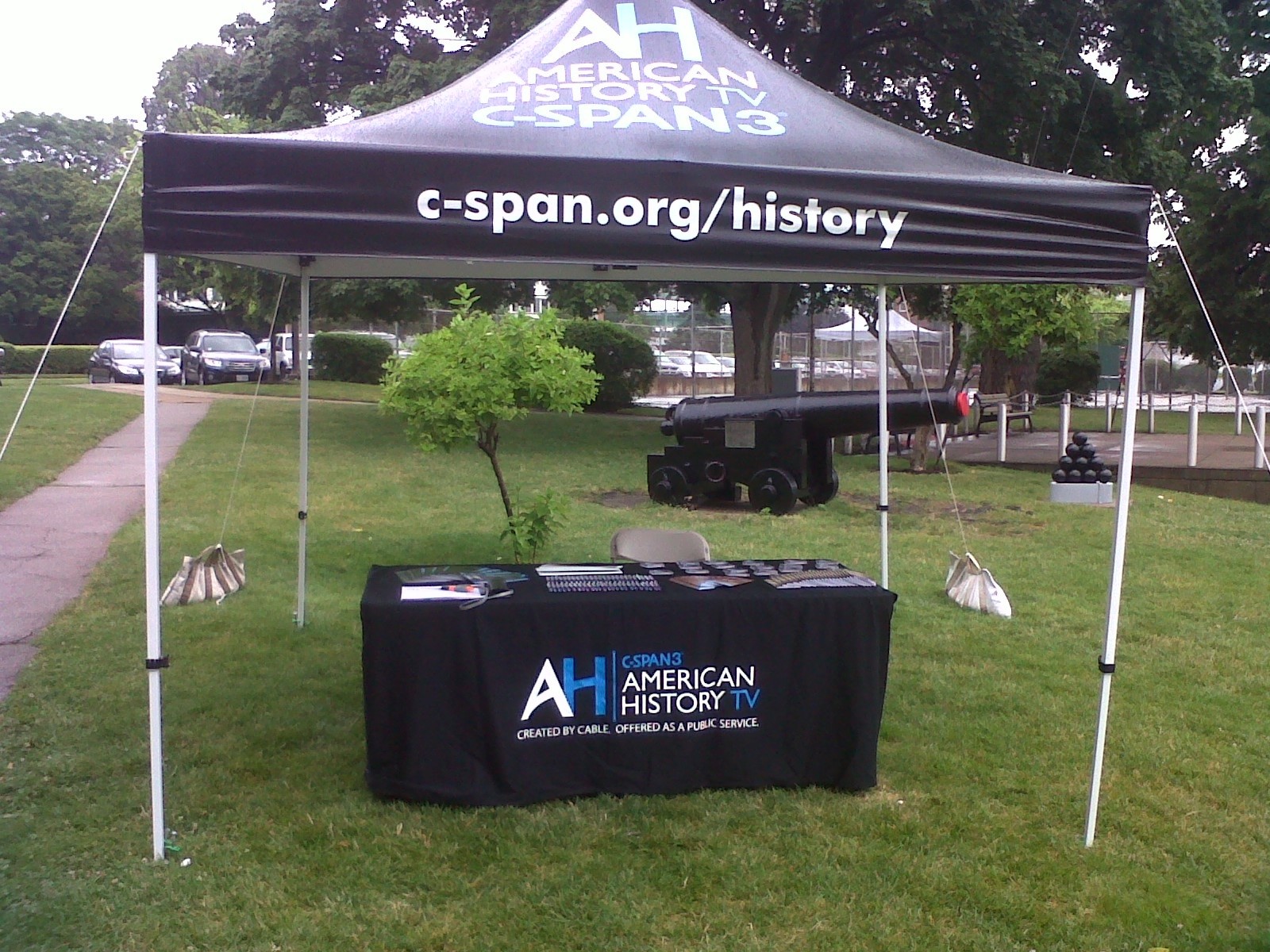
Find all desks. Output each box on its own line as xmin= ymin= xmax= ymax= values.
xmin=359 ymin=561 xmax=897 ymax=807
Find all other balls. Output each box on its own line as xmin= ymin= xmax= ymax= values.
xmin=1051 ymin=429 xmax=1113 ymax=484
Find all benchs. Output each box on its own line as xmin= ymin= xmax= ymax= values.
xmin=974 ymin=393 xmax=1034 ymax=439
xmin=864 ymin=429 xmax=915 ymax=455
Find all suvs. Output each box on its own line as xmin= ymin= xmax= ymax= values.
xmin=653 ymin=349 xmax=735 ymax=378
xmin=352 ymin=330 xmax=413 ymax=359
xmin=773 ymin=357 xmax=918 ymax=379
xmin=179 ymin=329 xmax=272 ymax=386
xmin=274 ymin=333 xmax=317 ymax=379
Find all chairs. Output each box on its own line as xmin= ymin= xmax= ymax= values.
xmin=610 ymin=528 xmax=710 ymax=563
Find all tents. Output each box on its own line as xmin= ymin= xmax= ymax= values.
xmin=141 ymin=0 xmax=1154 ymax=859
xmin=813 ymin=309 xmax=942 ymax=358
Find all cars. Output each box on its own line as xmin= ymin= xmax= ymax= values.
xmin=89 ymin=339 xmax=183 ymax=385
xmin=255 ymin=341 xmax=271 ymax=361
xmin=159 ymin=345 xmax=185 ymax=368
